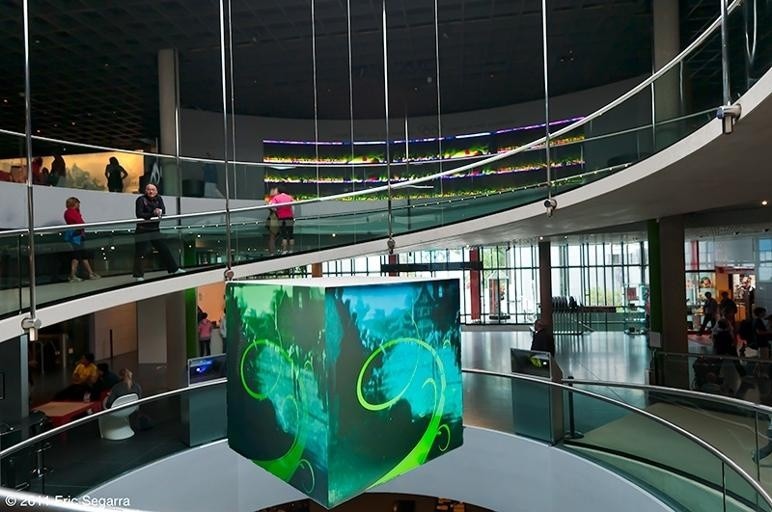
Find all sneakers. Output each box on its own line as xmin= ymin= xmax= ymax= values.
xmin=89 ymin=272 xmax=101 ymax=280
xmin=130 ymin=275 xmax=144 ymax=281
xmin=68 ymin=275 xmax=83 ymax=284
xmin=170 ymin=268 xmax=187 ymax=275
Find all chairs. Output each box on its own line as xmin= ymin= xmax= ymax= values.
xmin=98 ymin=392 xmax=140 ymax=441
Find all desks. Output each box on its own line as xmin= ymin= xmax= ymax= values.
xmin=33 ymin=401 xmax=96 ymax=441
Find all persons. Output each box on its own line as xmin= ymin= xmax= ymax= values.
xmin=219 ymin=308 xmax=228 ymax=353
xmin=63 ymin=196 xmax=104 ymax=284
xmin=198 ymin=311 xmax=213 ymax=357
xmin=268 ymin=184 xmax=296 ymax=255
xmin=53 ymin=350 xmax=99 ymax=400
xmin=739 ymin=272 xmax=754 ymax=304
xmin=131 ymin=182 xmax=186 ymax=282
xmin=263 ymin=186 xmax=279 ymax=258
xmin=700 ymin=276 xmax=711 ymax=288
xmin=104 ymin=156 xmax=129 ymax=193
xmin=530 ymin=320 xmax=554 ymax=356
xmin=105 ymin=366 xmax=145 ymax=413
xmin=31 ymin=153 xmax=66 ymax=187
xmin=696 ymin=290 xmax=771 ymax=377
